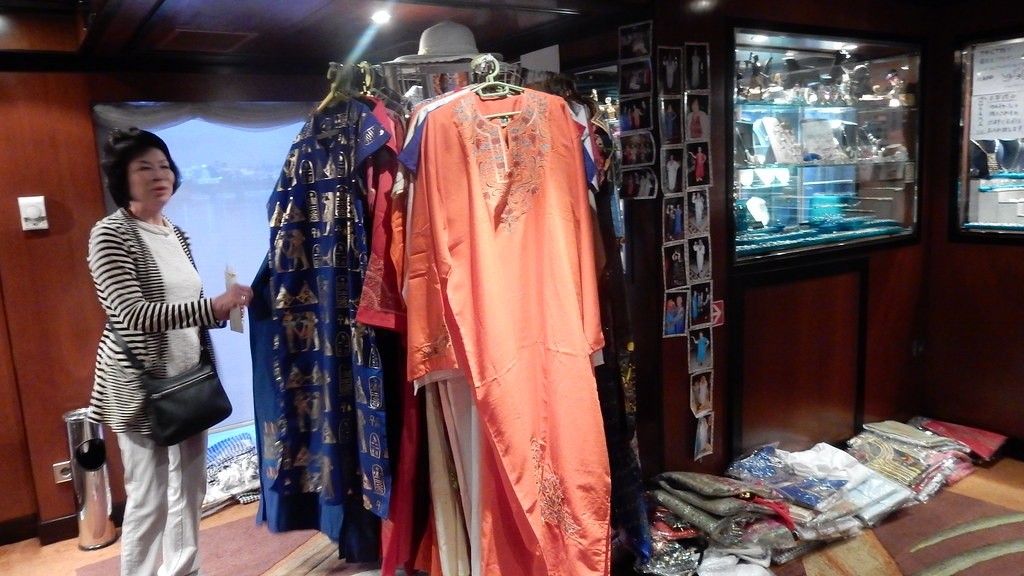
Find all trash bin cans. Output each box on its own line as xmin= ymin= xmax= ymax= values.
xmin=61 ymin=406 xmax=118 ymax=551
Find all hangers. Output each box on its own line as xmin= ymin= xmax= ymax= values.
xmin=314 ymin=61 xmax=393 ymax=140
xmin=463 ymin=53 xmax=524 ymax=119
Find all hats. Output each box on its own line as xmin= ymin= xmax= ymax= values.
xmin=379 ymin=19 xmax=505 ymax=66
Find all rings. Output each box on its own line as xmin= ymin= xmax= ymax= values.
xmin=241 ymin=294 xmax=246 ymax=300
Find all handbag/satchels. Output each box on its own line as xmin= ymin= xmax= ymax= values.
xmin=139 ymin=347 xmax=234 ymax=446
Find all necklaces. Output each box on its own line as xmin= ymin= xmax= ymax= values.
xmin=841 ymin=125 xmax=883 ymax=159
xmin=971 ymin=139 xmax=1022 ymax=174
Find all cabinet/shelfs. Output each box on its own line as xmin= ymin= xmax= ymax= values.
xmin=734 ymin=100 xmax=915 ymax=258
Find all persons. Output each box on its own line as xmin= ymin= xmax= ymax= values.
xmin=85 ymin=127 xmax=254 ymax=576
xmin=622 ymin=25 xmax=710 ymax=452
xmin=744 ymin=54 xmax=770 ymax=103
